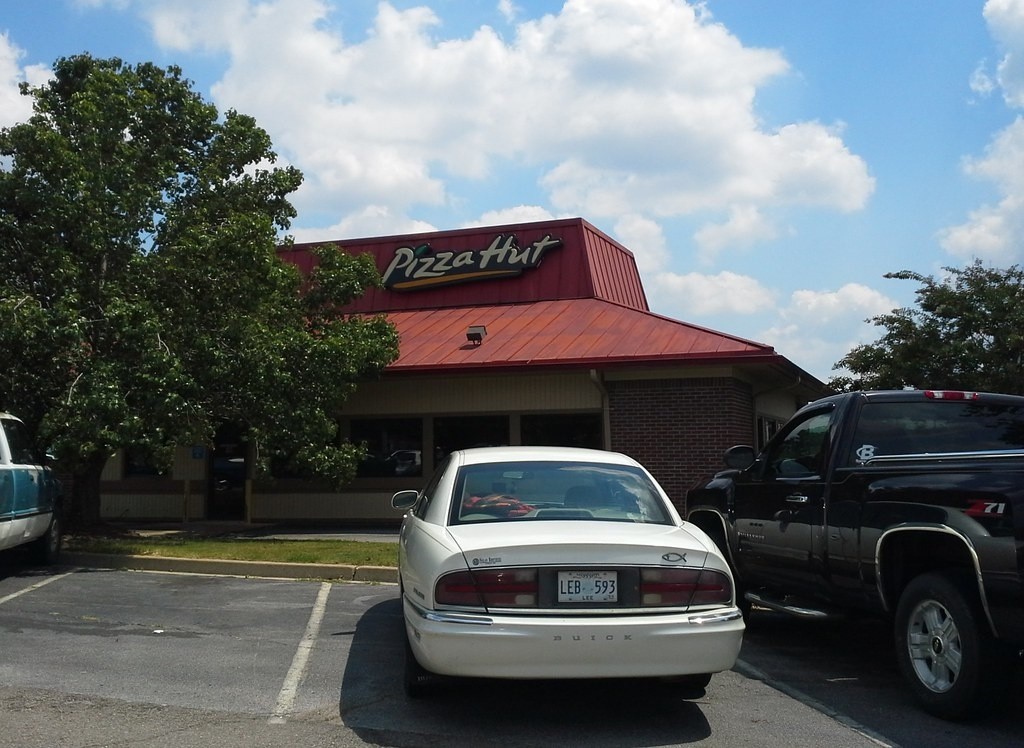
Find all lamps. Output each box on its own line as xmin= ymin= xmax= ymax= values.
xmin=466 ymin=325 xmax=488 ymax=346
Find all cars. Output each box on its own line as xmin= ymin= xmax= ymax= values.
xmin=390 ymin=447 xmax=746 ymax=705
xmin=209 ymin=456 xmax=247 ymax=514
xmin=353 ymin=448 xmax=423 ymax=478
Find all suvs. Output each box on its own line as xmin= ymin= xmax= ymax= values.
xmin=0 ymin=410 xmax=63 ymax=565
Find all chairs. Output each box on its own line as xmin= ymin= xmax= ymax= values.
xmin=564 ymin=486 xmax=604 ymax=508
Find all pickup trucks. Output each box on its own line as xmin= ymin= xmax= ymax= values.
xmin=683 ymin=390 xmax=1023 ymax=724
